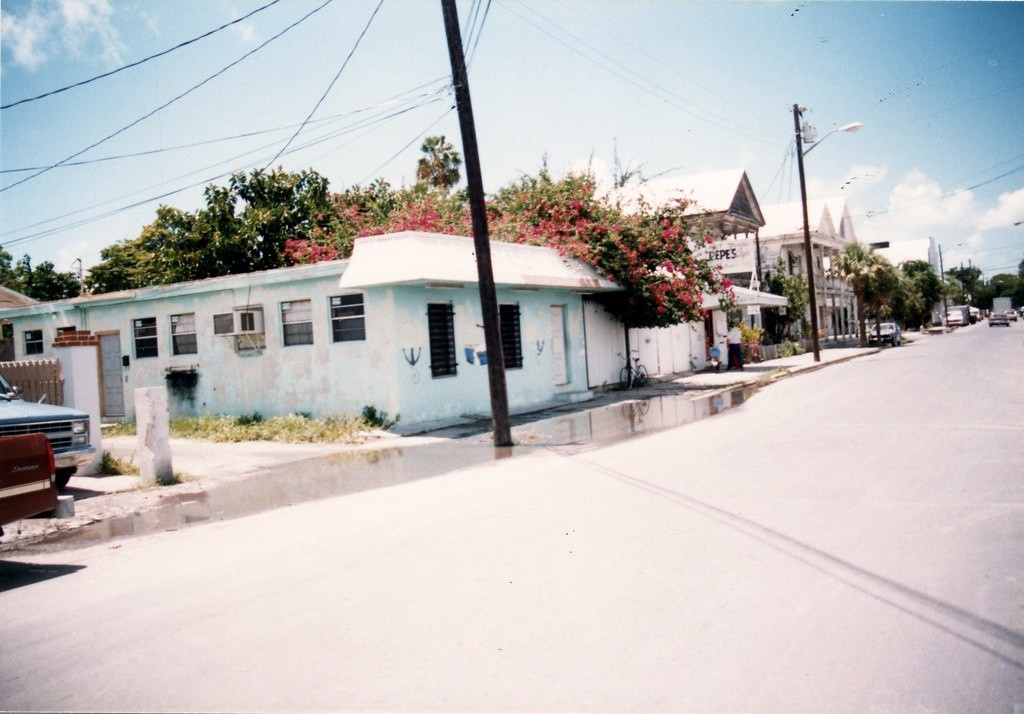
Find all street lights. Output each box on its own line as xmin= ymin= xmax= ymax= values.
xmin=940 ymin=243 xmax=962 ymax=327
xmin=798 ymin=122 xmax=863 ymax=362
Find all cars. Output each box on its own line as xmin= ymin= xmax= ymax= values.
xmin=1005 ymin=310 xmax=1017 ymax=322
xmin=1019 ymin=306 xmax=1024 ymax=318
xmin=989 ymin=311 xmax=1009 ymax=327
xmin=0 ymin=375 xmax=95 ymax=497
xmin=867 ymin=323 xmax=901 ymax=346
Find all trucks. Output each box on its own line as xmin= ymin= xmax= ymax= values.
xmin=941 ymin=306 xmax=970 ymax=326
xmin=971 ymin=309 xmax=984 ymax=321
xmin=993 ymin=297 xmax=1011 ymax=313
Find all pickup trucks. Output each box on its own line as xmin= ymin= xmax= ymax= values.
xmin=0 ymin=432 xmax=59 ymax=538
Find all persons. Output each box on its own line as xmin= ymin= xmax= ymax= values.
xmin=1020 ymin=306 xmax=1024 ymax=317
xmin=726 ymin=319 xmax=743 ymax=370
xmin=728 ymin=321 xmax=738 ymax=368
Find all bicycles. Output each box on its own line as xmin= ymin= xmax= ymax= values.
xmin=618 ymin=350 xmax=649 ymax=389
xmin=706 ymin=342 xmax=723 ymax=372
